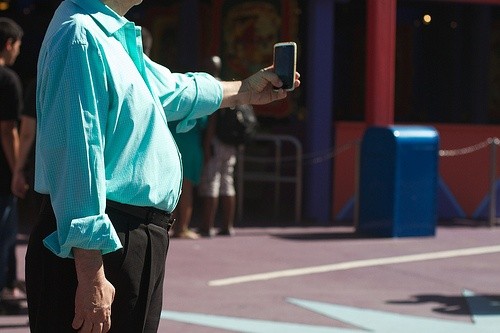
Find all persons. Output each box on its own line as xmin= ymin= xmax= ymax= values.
xmin=141 ymin=27 xmax=161 ymax=63
xmin=0 ymin=18 xmax=27 ymax=316
xmin=168 ymin=115 xmax=203 ymax=240
xmin=25 ymin=0 xmax=300 ymax=333
xmin=200 ymin=59 xmax=236 ymax=237
xmin=20 ymin=68 xmax=37 ymax=213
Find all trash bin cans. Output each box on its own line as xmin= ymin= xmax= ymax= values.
xmin=357 ymin=125 xmax=439 ymax=237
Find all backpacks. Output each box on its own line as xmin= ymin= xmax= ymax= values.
xmin=216 ymin=104 xmax=257 ymax=146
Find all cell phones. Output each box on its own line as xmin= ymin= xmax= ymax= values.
xmin=272 ymin=41 xmax=297 ymax=92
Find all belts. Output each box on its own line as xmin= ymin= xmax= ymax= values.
xmin=106 ymin=199 xmax=176 ymax=231
